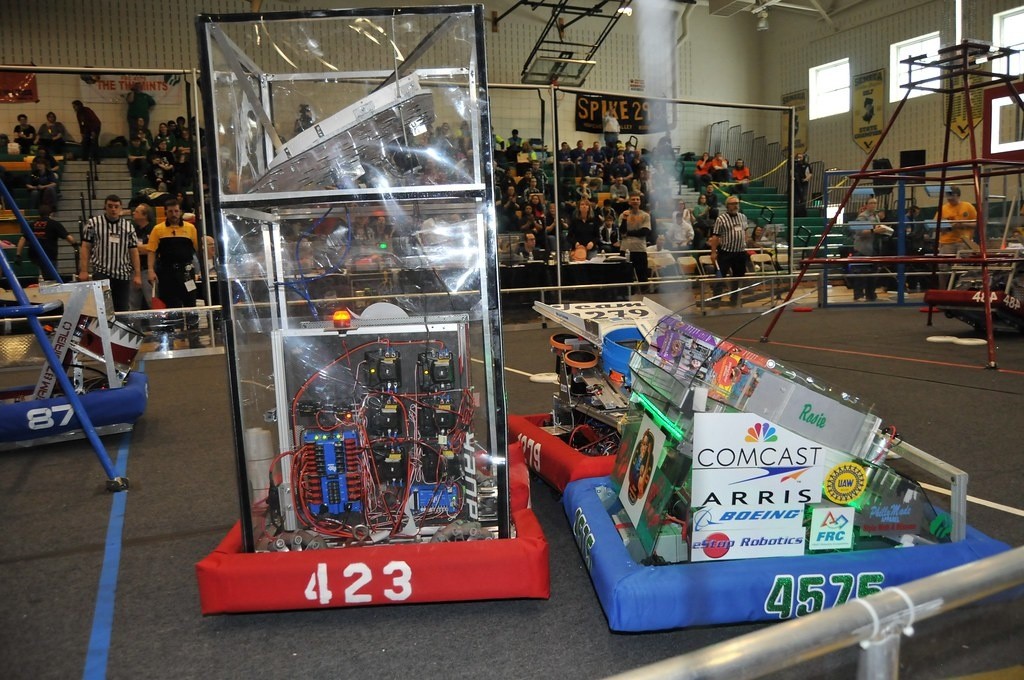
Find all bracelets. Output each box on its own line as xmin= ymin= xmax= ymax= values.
xmin=870 ymin=229 xmax=874 ymax=235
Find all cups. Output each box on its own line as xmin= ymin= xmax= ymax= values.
xmin=562 ymin=251 xmax=569 ymax=264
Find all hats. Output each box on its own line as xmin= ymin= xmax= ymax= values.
xmin=605 ymin=215 xmax=615 ymax=221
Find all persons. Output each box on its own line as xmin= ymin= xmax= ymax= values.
xmin=0 ymin=103 xmax=931 ymax=288
xmin=146 ymin=200 xmax=207 ymax=351
xmin=601 ymin=107 xmax=621 ymax=146
xmin=633 ymin=435 xmax=652 ymax=502
xmin=125 ymin=83 xmax=156 ymax=146
xmin=934 ymin=186 xmax=977 ymax=290
xmin=71 ymin=100 xmax=102 ymax=166
xmin=129 ymin=204 xmax=155 ymax=335
xmin=732 ymin=159 xmax=750 ymax=193
xmin=567 ymin=199 xmax=599 ymax=260
xmin=791 ymin=153 xmax=813 ymax=217
xmin=903 ymin=206 xmax=920 ymax=235
xmin=709 ymin=195 xmax=750 ymax=305
xmin=712 ymin=150 xmax=731 ymax=183
xmin=694 ymin=151 xmax=712 ymax=187
xmin=852 ymin=197 xmax=885 ymax=300
xmin=13 ymin=207 xmax=79 ymax=280
xmin=617 ymin=194 xmax=652 ymax=294
xmin=77 ymin=192 xmax=143 ymax=310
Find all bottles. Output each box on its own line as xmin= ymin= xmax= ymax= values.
xmin=625 ymin=249 xmax=630 ymax=262
xmin=38 ymin=275 xmax=44 ymax=292
xmin=548 ymin=254 xmax=554 ymax=265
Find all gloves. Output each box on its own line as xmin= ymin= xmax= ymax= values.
xmin=72 ymin=242 xmax=79 ymax=250
xmin=13 ymin=255 xmax=23 ymax=272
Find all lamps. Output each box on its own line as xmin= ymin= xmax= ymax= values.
xmin=757 ymin=10 xmax=769 ymax=32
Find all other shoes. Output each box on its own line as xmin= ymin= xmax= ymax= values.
xmin=156 ymin=341 xmax=175 ymax=351
xmin=856 ymin=298 xmax=867 ymax=302
xmin=189 ymin=340 xmax=205 ymax=348
xmin=712 ymin=301 xmax=721 ymax=309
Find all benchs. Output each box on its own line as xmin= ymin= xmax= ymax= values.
xmin=0 ymin=132 xmax=860 ymax=335
